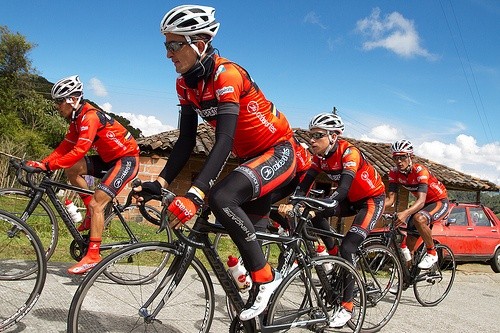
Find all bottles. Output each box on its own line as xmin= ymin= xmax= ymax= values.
xmin=316 ymin=245 xmax=333 ymax=273
xmin=401 ymin=241 xmax=411 ymax=263
xmin=225 ymin=255 xmax=252 ymax=293
xmin=65 ymin=198 xmax=83 ymax=223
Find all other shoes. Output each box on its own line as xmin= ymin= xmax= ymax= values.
xmin=77 ymin=216 xmax=91 ymax=231
xmin=388 ymin=282 xmax=398 ymax=294
xmin=324 ymin=251 xmax=340 ymax=280
xmin=329 ymin=304 xmax=356 ymax=328
xmin=68 ymin=255 xmax=102 ymax=274
xmin=417 ymin=251 xmax=438 ymax=269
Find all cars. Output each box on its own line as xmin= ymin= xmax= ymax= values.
xmin=355 ymin=198 xmax=500 ymax=273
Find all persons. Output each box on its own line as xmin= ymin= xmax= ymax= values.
xmin=384 ymin=139 xmax=449 ymax=293
xmin=26 ymin=74 xmax=140 ymax=273
xmin=271 ymin=132 xmax=311 ymax=204
xmin=279 ymin=112 xmax=386 ymax=329
xmin=132 ymin=5 xmax=297 ymax=321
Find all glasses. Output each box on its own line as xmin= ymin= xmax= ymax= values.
xmin=309 ymin=132 xmax=333 ymax=139
xmin=163 ymin=41 xmax=197 ymax=51
xmin=393 ymin=155 xmax=410 ymax=160
xmin=54 ymin=97 xmax=73 ymax=104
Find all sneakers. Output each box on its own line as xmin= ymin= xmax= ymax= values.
xmin=238 ymin=265 xmax=283 ymax=321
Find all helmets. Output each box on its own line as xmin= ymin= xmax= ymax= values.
xmin=391 ymin=139 xmax=413 ymax=155
xmin=308 ymin=112 xmax=345 ymax=135
xmin=159 ymin=5 xmax=220 ymax=38
xmin=51 ymin=73 xmax=83 ymax=99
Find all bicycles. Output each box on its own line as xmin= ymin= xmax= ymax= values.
xmin=226 ymin=202 xmax=404 ymax=332
xmin=67 ymin=178 xmax=367 ymax=333
xmin=350 ymin=212 xmax=457 ymax=310
xmin=0 ymin=152 xmax=171 ymax=285
xmin=0 ymin=209 xmax=48 ymax=332
